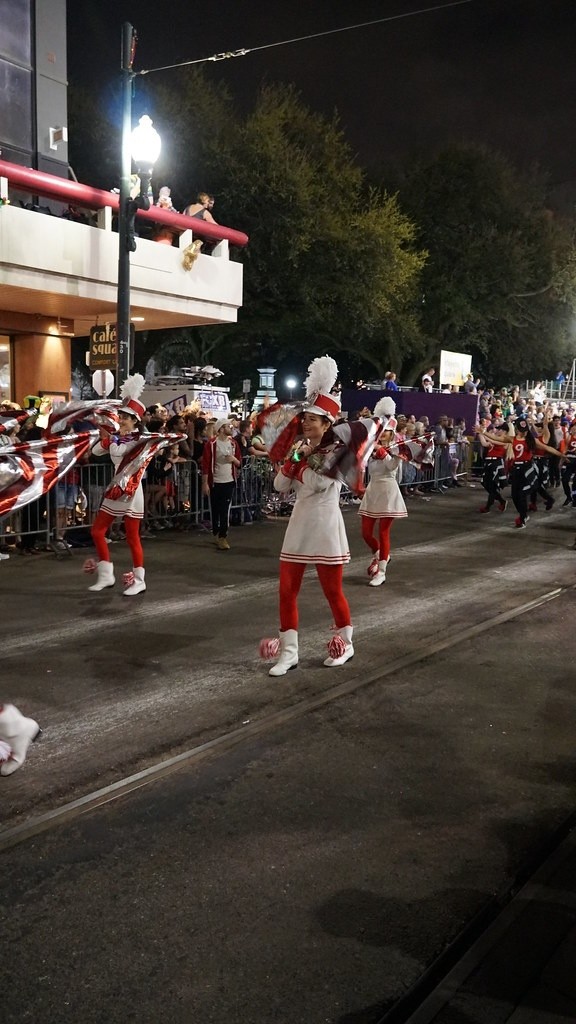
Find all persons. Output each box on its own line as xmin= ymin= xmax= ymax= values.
xmin=357 ymin=415 xmax=408 ymax=586
xmin=270 ymin=392 xmax=355 ymax=676
xmin=92 ymin=397 xmax=156 ymax=596
xmin=70 ymin=185 xmax=221 ymax=259
xmin=0 ymin=369 xmax=576 ymax=560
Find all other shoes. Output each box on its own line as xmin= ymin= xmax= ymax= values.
xmin=218 ymin=538 xmax=230 ymax=549
xmin=525 ymin=477 xmax=576 ymax=513
xmin=0 ymin=490 xmax=296 ymax=562
xmin=480 ymin=505 xmax=491 ymax=515
xmin=514 ymin=514 xmax=530 ymax=529
xmin=338 ymin=494 xmax=363 ymax=508
xmin=212 ymin=534 xmax=219 ymax=545
xmin=498 ymin=500 xmax=508 ymax=513
xmin=400 ymin=477 xmax=468 ymax=500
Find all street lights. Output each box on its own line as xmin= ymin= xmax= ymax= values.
xmin=113 ymin=112 xmax=160 ymax=401
xmin=286 ymin=380 xmax=299 ymax=400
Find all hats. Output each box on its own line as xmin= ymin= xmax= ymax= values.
xmin=301 ymin=354 xmax=342 ymax=425
xmin=373 ymin=396 xmax=398 ymax=433
xmin=117 ymin=372 xmax=146 ymax=421
xmin=214 ymin=417 xmax=234 ymax=432
xmin=482 ymin=401 xmax=576 ymax=434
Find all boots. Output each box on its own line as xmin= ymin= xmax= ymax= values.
xmin=260 ymin=629 xmax=299 ymax=677
xmin=0 ymin=704 xmax=43 ymax=778
xmin=368 ymin=560 xmax=387 ymax=586
xmin=323 ymin=625 xmax=355 ymax=667
xmin=82 ymin=559 xmax=116 ymax=592
xmin=122 ymin=566 xmax=146 ymax=596
xmin=368 ymin=549 xmax=391 ymax=576
